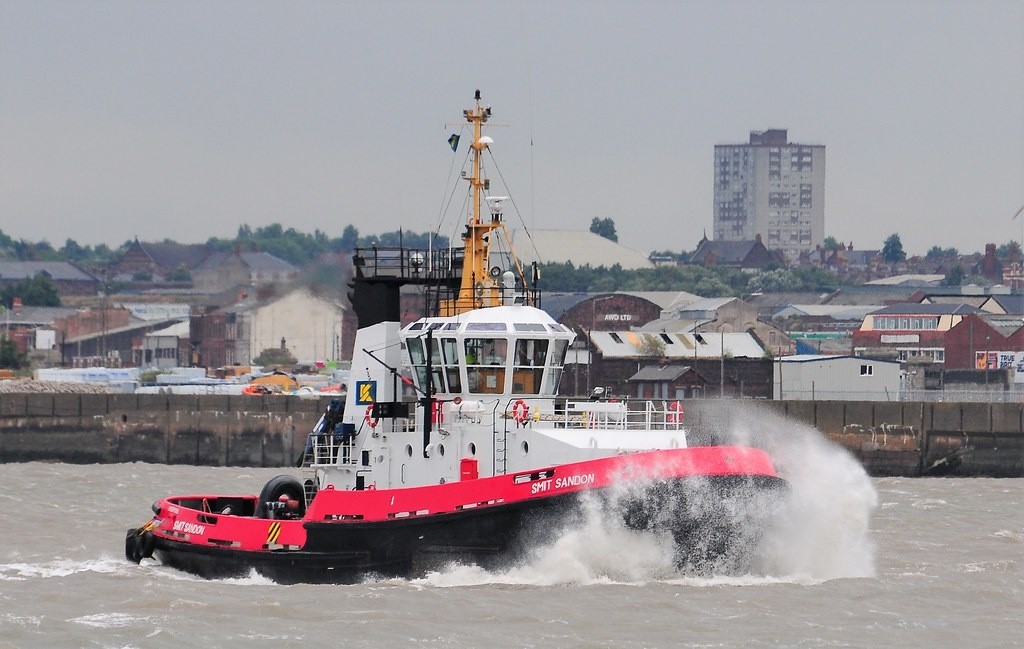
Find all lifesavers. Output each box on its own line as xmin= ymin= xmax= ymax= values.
xmin=669 ymin=402 xmax=684 ymax=424
xmin=366 ymin=404 xmax=380 ymax=427
xmin=513 ymin=400 xmax=529 ymax=423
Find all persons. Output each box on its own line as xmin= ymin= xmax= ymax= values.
xmin=465 ymin=348 xmax=480 ymax=365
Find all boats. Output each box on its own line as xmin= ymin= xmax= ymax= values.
xmin=124 ymin=88 xmax=792 ymax=587
